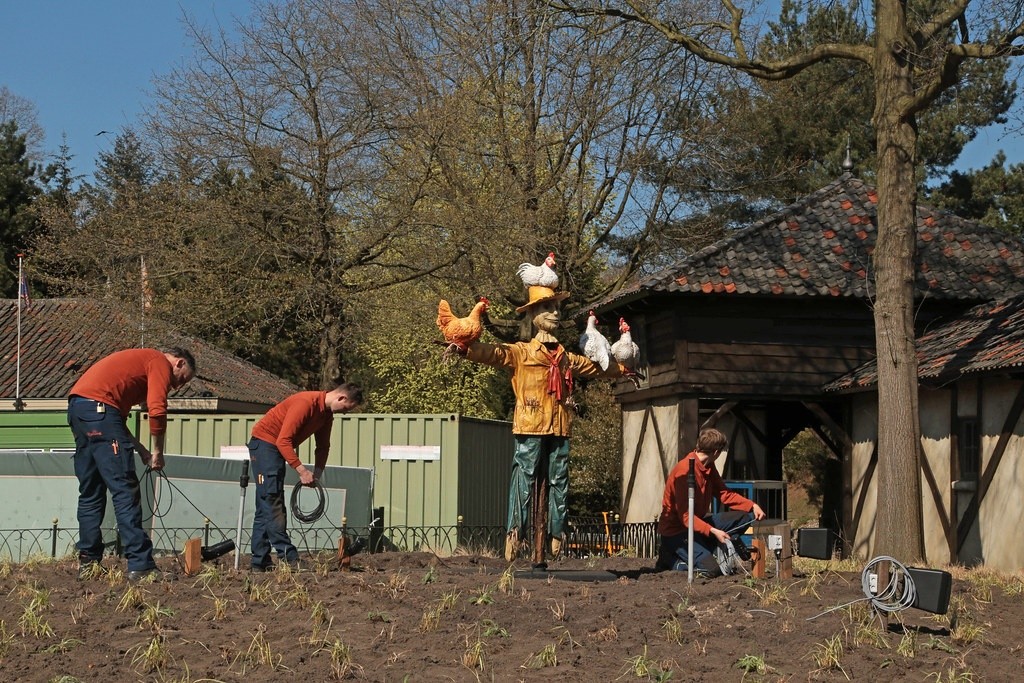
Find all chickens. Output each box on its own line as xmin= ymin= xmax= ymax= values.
xmin=578 ymin=311 xmax=612 ymax=372
xmin=436 ymin=296 xmax=492 ymax=351
xmin=515 ymin=252 xmax=559 ymax=290
xmin=611 ymin=317 xmax=640 ymax=373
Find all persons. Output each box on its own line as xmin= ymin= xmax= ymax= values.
xmin=456 ymin=284 xmax=637 ymax=557
xmin=66 ymin=347 xmax=197 ymax=586
xmin=244 ymin=382 xmax=365 ymax=574
xmin=656 ymin=428 xmax=767 ymax=579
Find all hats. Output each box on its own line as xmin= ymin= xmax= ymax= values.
xmin=517 ymin=286 xmax=570 ymax=313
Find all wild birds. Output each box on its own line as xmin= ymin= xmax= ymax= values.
xmin=93 ymin=130 xmax=114 ymax=137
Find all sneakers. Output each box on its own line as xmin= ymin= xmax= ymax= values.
xmin=282 ymin=560 xmax=320 ymax=572
xmin=78 ymin=565 xmax=110 ymax=581
xmin=128 ymin=568 xmax=178 ymax=582
xmin=249 ymin=565 xmax=277 ymax=573
